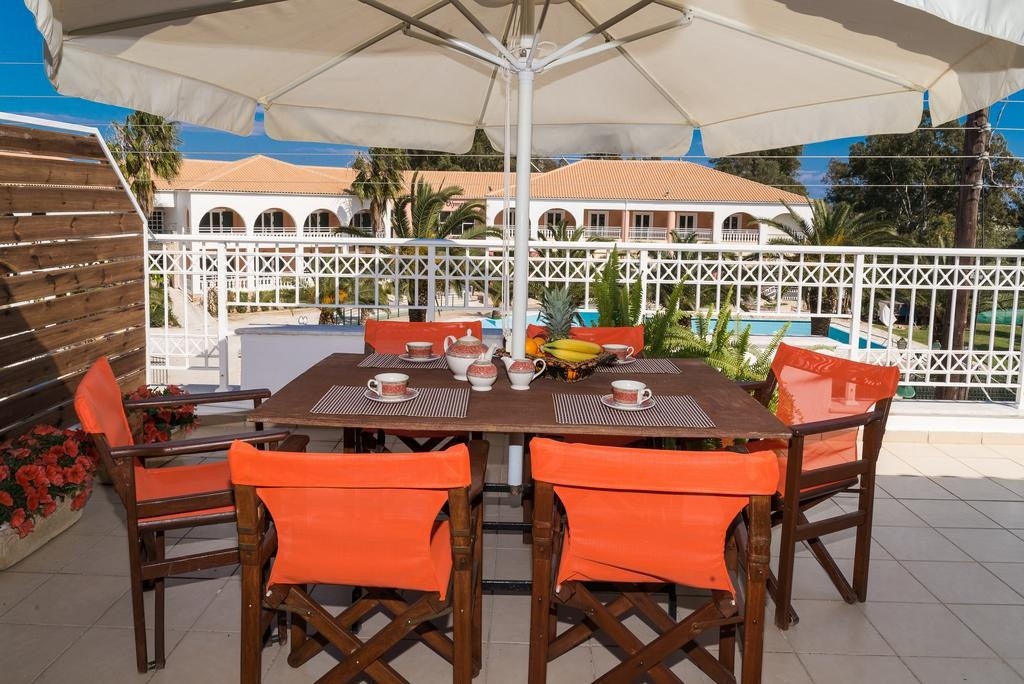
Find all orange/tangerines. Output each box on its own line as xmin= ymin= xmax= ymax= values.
xmin=525 ymin=336 xmax=545 ymax=355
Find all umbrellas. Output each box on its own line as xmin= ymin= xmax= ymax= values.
xmin=19 ymin=0 xmax=1024 ymax=360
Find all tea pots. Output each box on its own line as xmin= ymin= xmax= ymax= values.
xmin=444 ymin=329 xmax=498 ymax=381
xmin=466 ymin=353 xmax=498 ymax=392
xmin=500 ymin=356 xmax=546 ymax=391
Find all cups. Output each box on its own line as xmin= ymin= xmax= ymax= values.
xmin=367 ymin=372 xmax=409 ymax=399
xmin=602 ymin=343 xmax=634 ymax=361
xmin=405 ymin=341 xmax=433 ymax=359
xmin=611 ymin=380 xmax=652 ymax=407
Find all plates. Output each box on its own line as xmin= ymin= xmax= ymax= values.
xmin=364 ymin=388 xmax=420 ymax=403
xmin=601 ymin=394 xmax=655 ymax=411
xmin=398 ymin=353 xmax=441 ymax=362
xmin=604 ymin=358 xmax=637 ymax=365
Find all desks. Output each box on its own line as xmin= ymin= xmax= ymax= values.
xmin=247 ymin=351 xmax=789 ymax=624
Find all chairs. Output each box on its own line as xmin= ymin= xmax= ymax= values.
xmin=73 ymin=321 xmax=901 ymax=684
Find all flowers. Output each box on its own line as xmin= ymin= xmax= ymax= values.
xmin=0 ymin=425 xmax=97 ymax=538
xmin=74 ymin=385 xmax=202 ymax=467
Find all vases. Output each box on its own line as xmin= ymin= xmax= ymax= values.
xmin=144 ymin=424 xmax=188 ymax=468
xmin=92 ymin=409 xmax=142 ymax=485
xmin=1 ymin=491 xmax=83 ymax=572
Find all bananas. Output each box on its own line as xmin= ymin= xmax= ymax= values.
xmin=540 ymin=338 xmax=602 ymax=363
